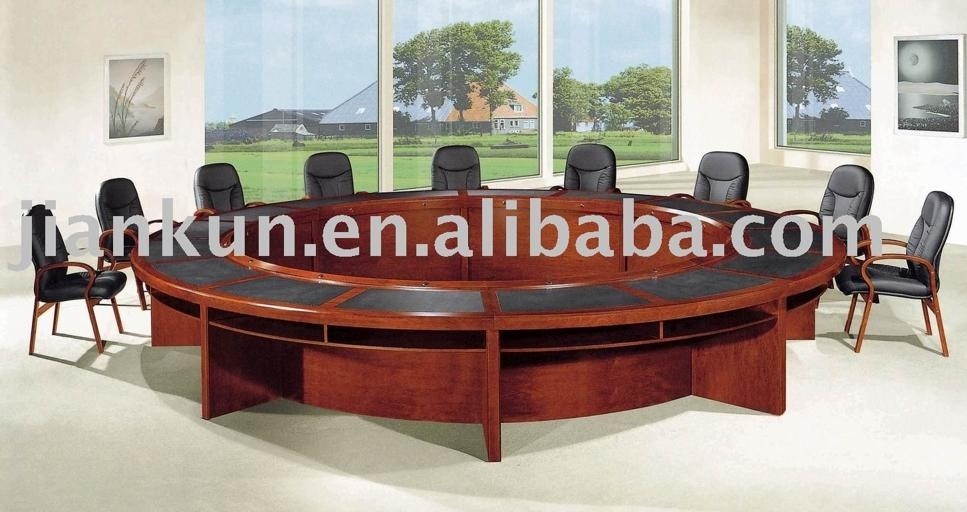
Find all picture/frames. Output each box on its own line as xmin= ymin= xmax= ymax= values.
xmin=103 ymin=51 xmax=170 ymax=146
xmin=892 ymin=35 xmax=966 ymax=138
xmin=133 ymin=189 xmax=847 ymax=465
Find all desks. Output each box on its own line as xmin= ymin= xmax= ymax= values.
xmin=133 ymin=189 xmax=847 ymax=465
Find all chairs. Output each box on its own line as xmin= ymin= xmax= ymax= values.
xmin=23 ymin=143 xmax=954 ymax=361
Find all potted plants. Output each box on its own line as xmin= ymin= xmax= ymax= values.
xmin=103 ymin=51 xmax=170 ymax=146
xmin=892 ymin=35 xmax=966 ymax=138
